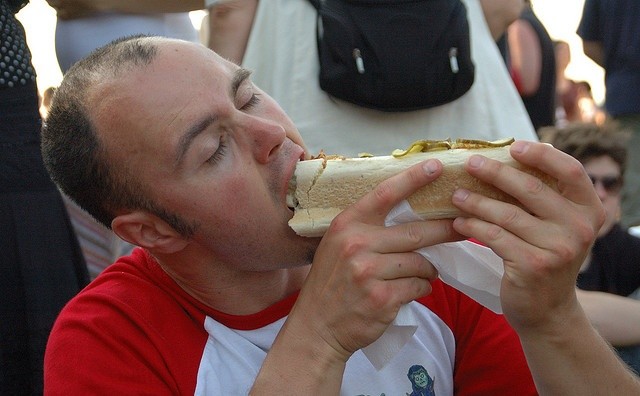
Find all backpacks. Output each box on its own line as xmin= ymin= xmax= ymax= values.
xmin=309 ymin=1 xmax=477 ymax=115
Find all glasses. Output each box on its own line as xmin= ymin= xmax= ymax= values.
xmin=588 ymin=173 xmax=626 ymax=197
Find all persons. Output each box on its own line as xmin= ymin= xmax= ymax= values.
xmin=0 ymin=0 xmax=92 ymax=396
xmin=42 ymin=32 xmax=640 ymax=396
xmin=496 ymin=0 xmax=556 ymax=129
xmin=546 ymin=122 xmax=639 ymax=376
xmin=550 ymin=40 xmax=603 ymax=122
xmin=576 ymin=0 xmax=639 ymax=122
xmin=44 ymin=1 xmax=202 ymax=280
xmin=207 ymin=0 xmax=539 ymax=163
